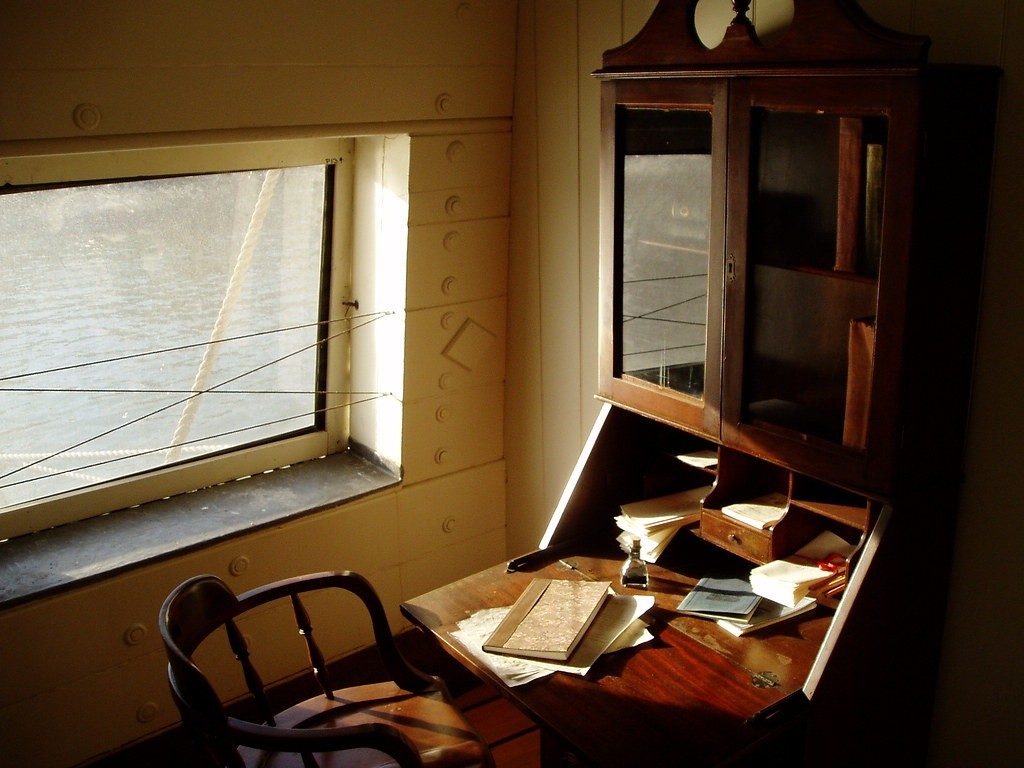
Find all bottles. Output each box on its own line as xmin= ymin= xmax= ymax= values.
xmin=619 ymin=543 xmax=649 ymax=590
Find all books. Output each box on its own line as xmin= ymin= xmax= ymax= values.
xmin=482 ymin=578 xmax=613 ymax=666
xmin=834 ymin=117 xmax=883 ymax=275
xmin=715 ymin=597 xmax=817 ymax=638
xmin=843 ymin=315 xmax=874 ymax=450
xmin=675 ymin=577 xmax=763 ymax=625
xmin=721 ymin=493 xmax=788 ymax=530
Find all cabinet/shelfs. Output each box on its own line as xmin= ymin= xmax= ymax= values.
xmin=401 ymin=0 xmax=1012 ymax=768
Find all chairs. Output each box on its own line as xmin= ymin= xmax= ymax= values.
xmin=159 ymin=570 xmax=496 ymax=768
